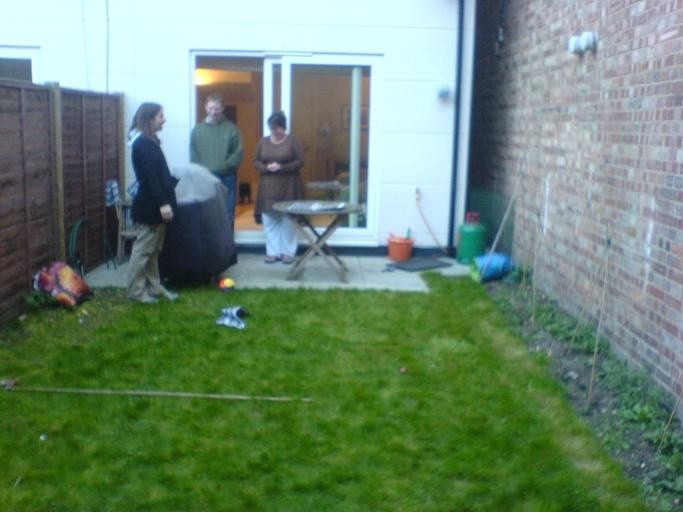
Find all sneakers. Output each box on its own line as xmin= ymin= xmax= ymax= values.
xmin=264 ymin=254 xmax=294 ymax=265
xmin=130 ymin=288 xmax=179 ymax=304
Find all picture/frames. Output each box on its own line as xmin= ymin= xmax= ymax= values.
xmin=341 ymin=103 xmax=368 ymax=132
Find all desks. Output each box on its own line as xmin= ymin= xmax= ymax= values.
xmin=272 ymin=199 xmax=362 ymax=283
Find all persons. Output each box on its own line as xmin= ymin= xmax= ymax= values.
xmin=188 ymin=92 xmax=244 ymax=230
xmin=254 ymin=110 xmax=304 ymax=265
xmin=124 ymin=102 xmax=179 ymax=303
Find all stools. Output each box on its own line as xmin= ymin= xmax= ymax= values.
xmin=304 ymin=180 xmax=340 ymax=218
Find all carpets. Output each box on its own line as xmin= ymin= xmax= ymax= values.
xmin=385 ymin=255 xmax=453 ymax=272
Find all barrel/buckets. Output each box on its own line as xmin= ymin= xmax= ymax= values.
xmin=388 ymin=237 xmax=413 ymax=262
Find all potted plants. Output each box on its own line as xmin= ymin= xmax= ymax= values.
xmin=387 ymin=226 xmax=414 ymax=262
